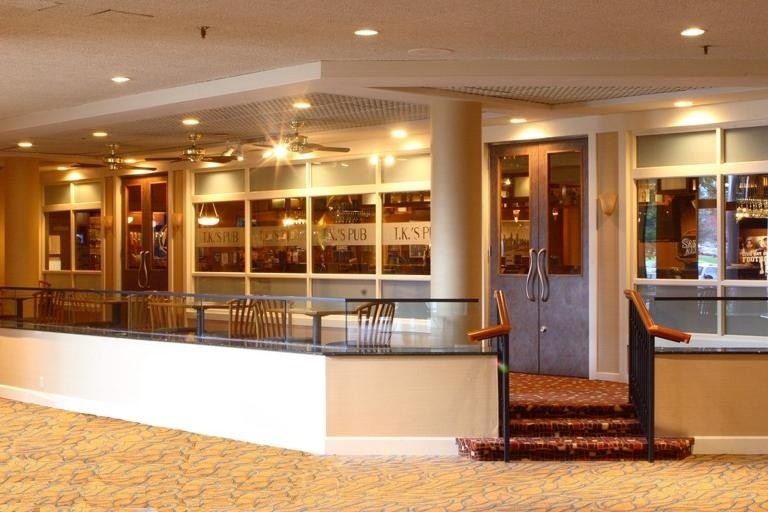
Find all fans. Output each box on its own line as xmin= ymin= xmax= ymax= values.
xmin=253 ymin=120 xmax=352 ymax=156
xmin=144 ymin=132 xmax=240 ymax=165
xmin=69 ymin=143 xmax=158 ymax=173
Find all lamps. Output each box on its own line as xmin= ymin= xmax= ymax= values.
xmin=198 ymin=203 xmax=220 ymax=226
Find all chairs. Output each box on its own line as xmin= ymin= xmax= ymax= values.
xmin=15 ymin=280 xmax=158 ymax=353
xmin=249 ymin=297 xmax=313 ymax=353
xmin=226 ymin=297 xmax=257 ymax=349
xmin=322 ymin=300 xmax=393 ymax=354
xmin=141 ymin=292 xmax=194 ymax=342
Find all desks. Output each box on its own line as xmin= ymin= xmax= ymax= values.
xmin=148 ymin=302 xmax=253 ymax=345
xmin=0 ymin=294 xmax=36 ymax=322
xmin=265 ymin=307 xmax=367 ymax=353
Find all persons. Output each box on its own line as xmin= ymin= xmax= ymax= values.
xmin=756 ymin=237 xmax=767 ymax=256
xmin=740 ymin=239 xmax=756 ymax=257
